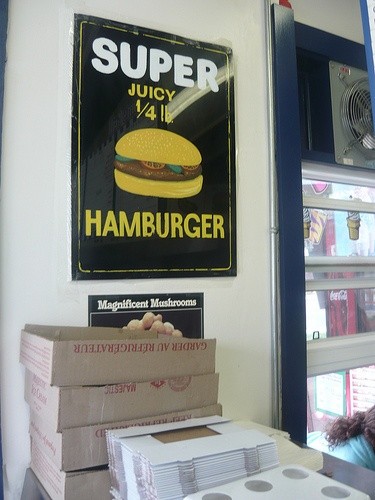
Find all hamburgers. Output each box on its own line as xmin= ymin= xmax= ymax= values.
xmin=114 ymin=128 xmax=203 ymax=199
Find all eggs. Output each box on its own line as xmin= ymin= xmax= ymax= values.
xmin=122 ymin=312 xmax=183 ymax=336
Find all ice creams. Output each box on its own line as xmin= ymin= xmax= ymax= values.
xmin=303 ymin=208 xmax=311 ymax=239
xmin=347 ymin=211 xmax=362 ymax=240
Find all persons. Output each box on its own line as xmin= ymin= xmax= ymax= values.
xmin=309 ymin=404 xmax=375 ymax=472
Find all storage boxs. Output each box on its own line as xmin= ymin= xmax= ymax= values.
xmin=29 ymin=403 xmax=222 ymax=472
xmin=29 ymin=438 xmax=113 ymax=500
xmin=19 ymin=325 xmax=217 ymax=387
xmin=23 ymin=367 xmax=220 ymax=432
